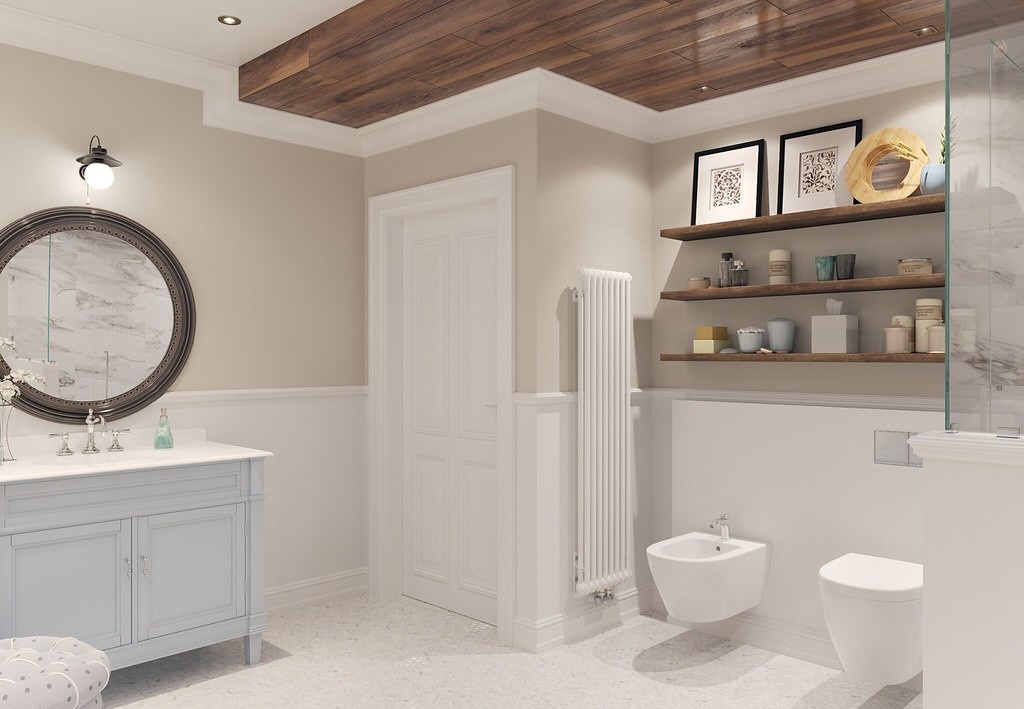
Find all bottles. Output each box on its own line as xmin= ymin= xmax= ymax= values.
xmin=155 ymin=408 xmax=174 ymax=449
xmin=731 ymin=260 xmax=748 ymax=286
xmin=718 ymin=253 xmax=734 ymax=288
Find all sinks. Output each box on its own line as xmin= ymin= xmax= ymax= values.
xmin=646 ymin=531 xmax=773 ymax=623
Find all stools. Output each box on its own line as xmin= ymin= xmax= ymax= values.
xmin=0 ymin=636 xmax=109 ymax=709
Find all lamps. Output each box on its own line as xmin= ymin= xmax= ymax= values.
xmin=77 ymin=135 xmax=121 ymax=191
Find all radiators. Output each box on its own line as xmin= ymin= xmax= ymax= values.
xmin=572 ymin=267 xmax=633 ymax=595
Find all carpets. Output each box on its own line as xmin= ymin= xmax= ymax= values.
xmin=100 ymin=636 xmax=293 ymax=709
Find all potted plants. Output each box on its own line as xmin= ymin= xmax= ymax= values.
xmin=894 ymin=113 xmax=977 ymax=193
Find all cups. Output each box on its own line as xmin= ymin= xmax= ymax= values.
xmin=768 ymin=250 xmax=792 ymax=285
xmin=835 ymin=254 xmax=856 ymax=280
xmin=815 ymin=256 xmax=836 ymax=281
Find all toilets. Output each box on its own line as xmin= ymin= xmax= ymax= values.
xmin=818 ymin=552 xmax=923 ymax=685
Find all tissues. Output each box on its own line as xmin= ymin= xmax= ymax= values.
xmin=810 ymin=297 xmax=860 ymax=354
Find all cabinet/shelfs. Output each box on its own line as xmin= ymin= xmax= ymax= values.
xmin=660 ymin=187 xmax=1015 ymax=364
xmin=0 ymin=429 xmax=273 ymax=667
xmin=910 ymin=430 xmax=1024 ymax=708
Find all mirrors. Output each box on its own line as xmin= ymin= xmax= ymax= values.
xmin=0 ymin=207 xmax=196 ymax=425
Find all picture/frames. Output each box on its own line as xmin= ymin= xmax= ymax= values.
xmin=691 ymin=139 xmax=763 ymax=225
xmin=777 ymin=119 xmax=863 ymax=215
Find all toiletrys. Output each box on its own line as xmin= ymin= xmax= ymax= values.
xmin=883 ymin=297 xmax=976 ymax=355
xmin=692 ymin=317 xmax=797 ymax=356
xmin=686 ymin=249 xmax=934 ymax=291
xmin=153 ymin=407 xmax=174 ymax=449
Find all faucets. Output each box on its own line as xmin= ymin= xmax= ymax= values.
xmin=708 ymin=512 xmax=730 ymax=540
xmin=79 ymin=407 xmax=108 ymax=454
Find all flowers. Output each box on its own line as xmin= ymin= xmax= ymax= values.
xmin=1 ymin=335 xmax=46 ymax=401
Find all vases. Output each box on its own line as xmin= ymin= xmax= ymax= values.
xmin=0 ymin=405 xmax=16 ymax=461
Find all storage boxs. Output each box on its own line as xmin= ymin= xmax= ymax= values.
xmin=811 ymin=315 xmax=858 ymax=354
xmin=693 ymin=326 xmax=729 ymax=353
